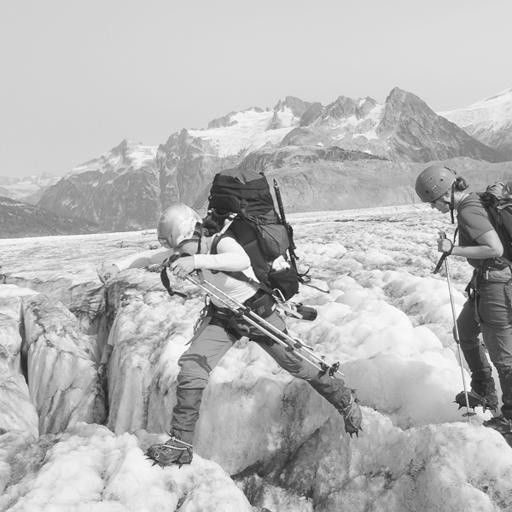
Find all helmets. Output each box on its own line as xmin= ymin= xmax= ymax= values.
xmin=157 ymin=204 xmax=202 ymax=249
xmin=415 ymin=163 xmax=457 ymax=203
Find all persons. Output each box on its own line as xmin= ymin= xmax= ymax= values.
xmin=148 ymin=203 xmax=362 ymax=464
xmin=415 ymin=165 xmax=512 ymax=434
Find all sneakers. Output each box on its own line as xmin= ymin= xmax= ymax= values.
xmin=144 ymin=437 xmax=194 ymax=469
xmin=455 ymin=389 xmax=498 ymax=413
xmin=338 ymin=388 xmax=362 ymax=438
xmin=482 ymin=415 xmax=512 ymax=434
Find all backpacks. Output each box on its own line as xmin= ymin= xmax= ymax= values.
xmin=204 ymin=167 xmax=299 ymax=301
xmin=453 ymin=180 xmax=512 ymax=270
